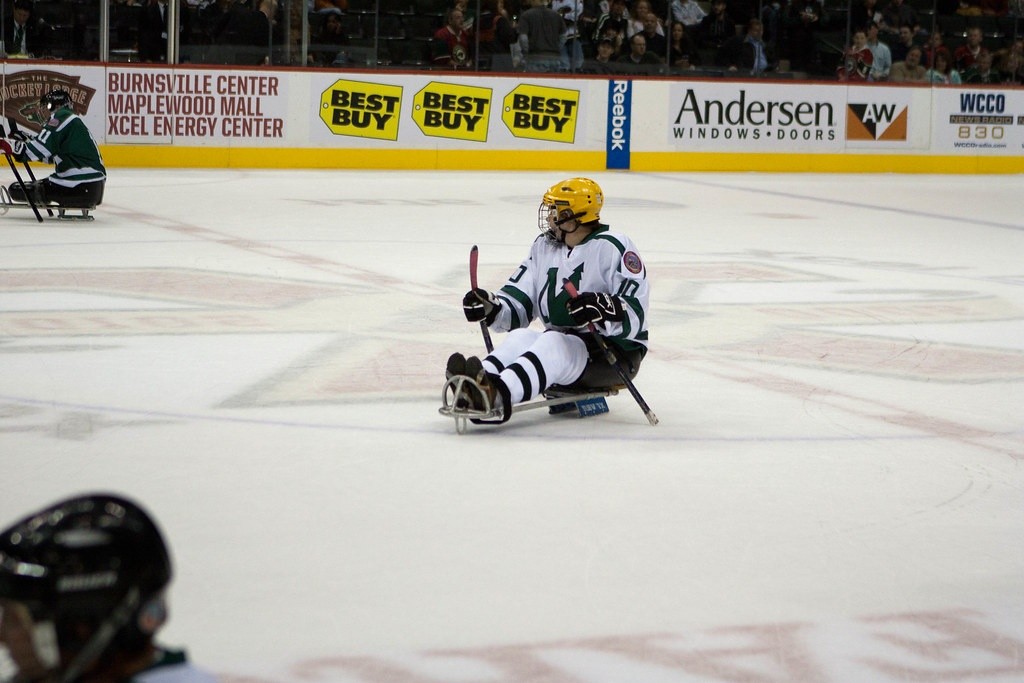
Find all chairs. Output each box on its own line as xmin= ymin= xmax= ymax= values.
xmin=0 ymin=0 xmax=1023 ymax=83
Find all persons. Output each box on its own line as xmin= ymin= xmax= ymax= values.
xmin=0 ymin=0 xmax=1024 ymax=86
xmin=0 ymin=90 xmax=106 ymax=204
xmin=0 ymin=494 xmax=214 ymax=683
xmin=446 ymin=177 xmax=650 ymax=411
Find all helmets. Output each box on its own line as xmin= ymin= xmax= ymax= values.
xmin=0 ymin=493 xmax=186 ymax=683
xmin=538 ymin=177 xmax=604 ymax=241
xmin=40 ymin=89 xmax=73 ymax=111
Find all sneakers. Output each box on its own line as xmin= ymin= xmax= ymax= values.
xmin=465 ymin=356 xmax=495 ymax=410
xmin=446 ymin=353 xmax=467 ymax=408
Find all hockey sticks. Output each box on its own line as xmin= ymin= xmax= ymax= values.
xmin=7 ymin=117 xmax=55 ymax=218
xmin=561 ymin=277 xmax=659 ymax=427
xmin=470 ymin=245 xmax=494 ymax=354
xmin=0 ymin=124 xmax=43 ymax=223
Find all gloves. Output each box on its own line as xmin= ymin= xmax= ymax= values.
xmin=8 ymin=131 xmax=33 ymax=143
xmin=0 ymin=138 xmax=23 ymax=154
xmin=566 ymin=292 xmax=624 ymax=329
xmin=463 ymin=289 xmax=502 ymax=326
